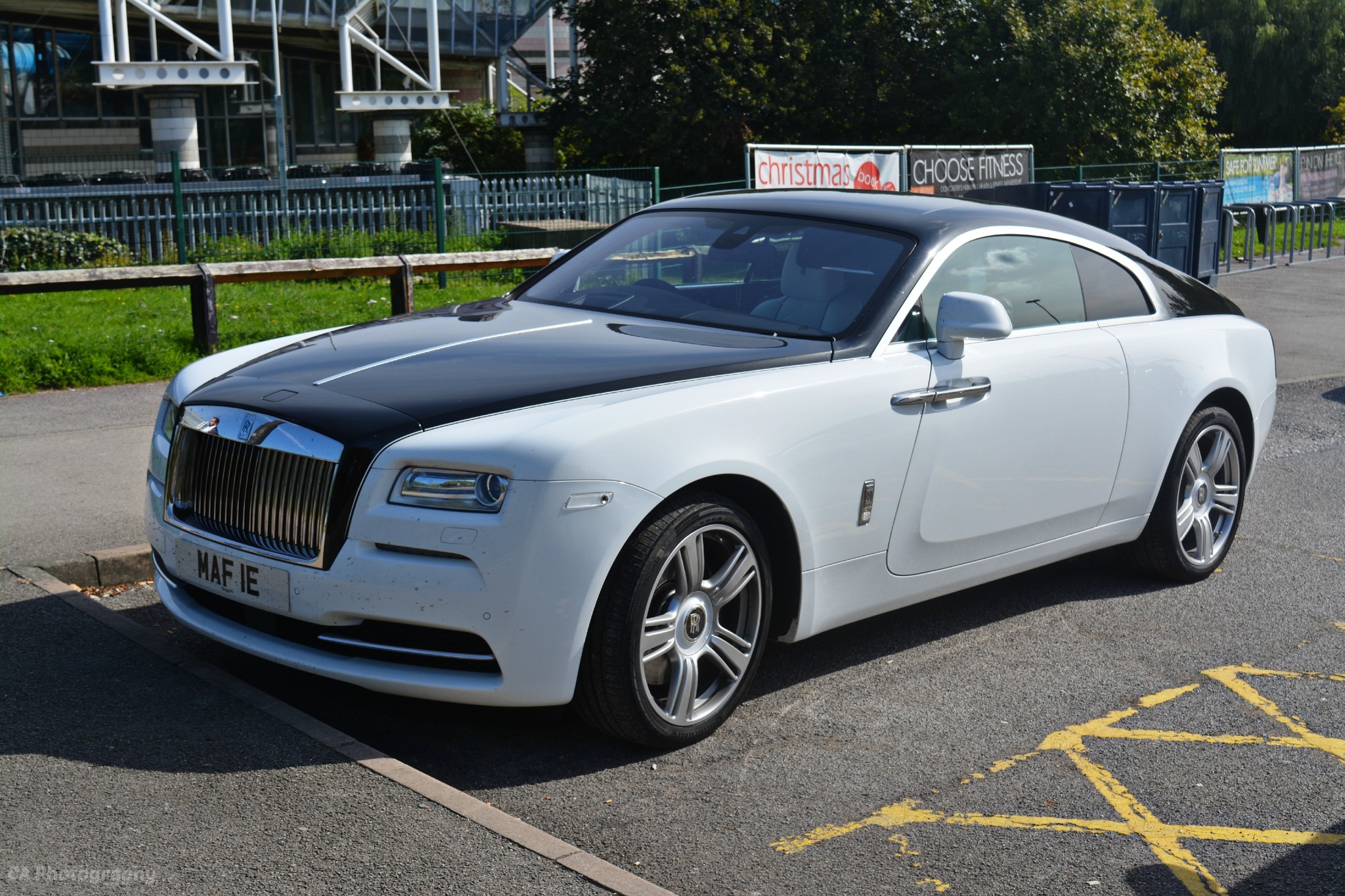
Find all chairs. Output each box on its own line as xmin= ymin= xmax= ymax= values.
xmin=749 ymin=249 xmax=860 ymax=337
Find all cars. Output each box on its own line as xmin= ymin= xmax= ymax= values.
xmin=144 ymin=184 xmax=1273 ymax=753
